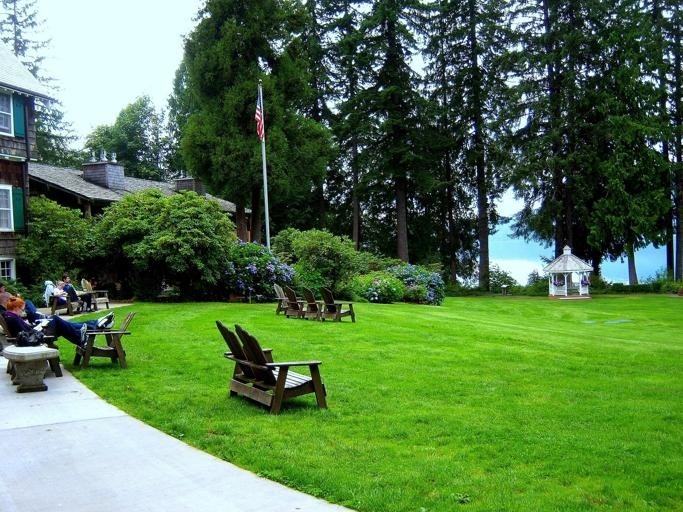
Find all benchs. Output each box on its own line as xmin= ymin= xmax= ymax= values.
xmin=216 ymin=320 xmax=327 ymax=415
xmin=2 ymin=343 xmax=60 ymax=393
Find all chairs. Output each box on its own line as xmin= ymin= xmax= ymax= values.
xmin=73 ymin=312 xmax=136 ymax=368
xmin=45 ymin=281 xmax=84 ymax=316
xmin=0 ymin=314 xmax=62 ymax=377
xmin=274 ymin=284 xmax=355 ymax=322
xmin=81 ymin=278 xmax=109 ymax=311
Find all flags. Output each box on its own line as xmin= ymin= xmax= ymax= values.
xmin=254 ymin=88 xmax=264 ymax=141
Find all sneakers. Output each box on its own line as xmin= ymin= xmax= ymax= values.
xmin=80 ymin=324 xmax=89 ymax=352
xmin=99 ymin=312 xmax=115 ymax=328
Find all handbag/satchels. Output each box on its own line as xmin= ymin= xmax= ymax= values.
xmin=16 ymin=330 xmax=44 ymax=346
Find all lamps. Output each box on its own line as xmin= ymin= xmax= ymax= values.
xmin=89 ymin=149 xmax=117 ymax=164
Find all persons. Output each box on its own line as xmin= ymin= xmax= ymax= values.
xmin=0 ymin=274 xmax=116 ymax=350
